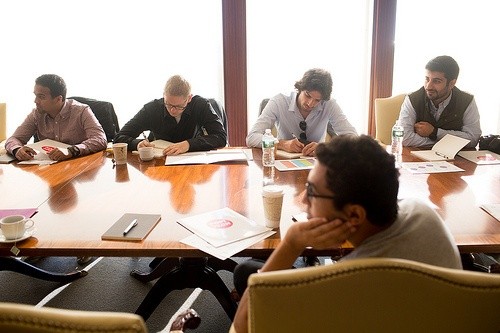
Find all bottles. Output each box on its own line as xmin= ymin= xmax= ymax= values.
xmin=262 ymin=129 xmax=275 ymax=168
xmin=391 ymin=120 xmax=404 ymax=156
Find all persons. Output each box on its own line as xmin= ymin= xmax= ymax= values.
xmin=4 ymin=74 xmax=108 ymax=163
xmin=245 ymin=68 xmax=360 ymax=157
xmin=112 ymin=74 xmax=227 ymax=157
xmin=233 ymin=133 xmax=466 ymax=333
xmin=392 ymin=55 xmax=481 ymax=149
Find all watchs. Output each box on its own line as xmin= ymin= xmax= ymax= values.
xmin=67 ymin=145 xmax=80 ymax=158
xmin=429 ymin=127 xmax=438 ymax=141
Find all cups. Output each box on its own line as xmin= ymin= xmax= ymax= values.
xmin=139 ymin=146 xmax=154 ymax=160
xmin=0 ymin=215 xmax=35 ymax=239
xmin=262 ymin=185 xmax=285 ymax=230
xmin=112 ymin=142 xmax=128 ymax=166
xmin=273 ymin=138 xmax=279 ymax=157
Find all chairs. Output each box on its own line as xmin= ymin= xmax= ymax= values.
xmin=34 ymin=97 xmax=120 ymax=144
xmin=248 ymin=256 xmax=500 ymax=333
xmin=374 ymin=93 xmax=407 ymax=145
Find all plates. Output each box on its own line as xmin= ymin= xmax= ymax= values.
xmin=0 ymin=225 xmax=35 ymax=242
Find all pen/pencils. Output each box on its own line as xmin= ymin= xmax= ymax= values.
xmin=291 ymin=216 xmax=298 ymax=223
xmin=292 ymin=132 xmax=298 ymax=141
xmin=123 ymin=219 xmax=138 ymax=235
xmin=435 ymin=152 xmax=448 ymax=159
xmin=30 ymin=151 xmax=34 ymax=157
xmin=143 ymin=133 xmax=149 ymax=143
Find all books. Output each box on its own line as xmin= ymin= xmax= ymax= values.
xmin=101 ymin=212 xmax=162 ymax=241
xmin=276 ymin=148 xmax=304 ymax=158
xmin=409 ymin=133 xmax=471 ymax=161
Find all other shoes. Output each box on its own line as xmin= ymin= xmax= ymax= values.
xmin=21 ymin=257 xmax=40 ymax=263
xmin=77 ymin=256 xmax=92 ymax=265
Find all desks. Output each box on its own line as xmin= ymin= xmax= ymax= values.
xmin=0 ymin=147 xmax=500 ymax=323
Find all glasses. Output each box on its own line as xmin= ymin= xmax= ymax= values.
xmin=299 ymin=121 xmax=307 ymax=142
xmin=162 ymin=94 xmax=187 ymax=109
xmin=305 ymin=183 xmax=349 ymax=204
